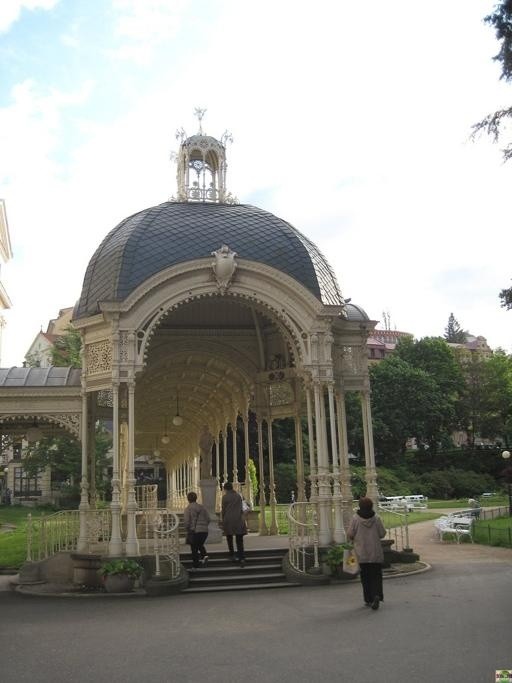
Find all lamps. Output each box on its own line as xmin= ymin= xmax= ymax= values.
xmin=148 ymin=393 xmax=183 ymax=465
xmin=24 ymin=417 xmax=44 ymax=443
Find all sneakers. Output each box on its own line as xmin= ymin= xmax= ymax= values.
xmin=364 ymin=600 xmax=371 ymax=606
xmin=202 ymin=555 xmax=209 ymax=568
xmin=371 ymin=593 xmax=381 ymax=610
xmin=193 ymin=560 xmax=202 ymax=569
xmin=230 ymin=554 xmax=237 ymax=563
xmin=239 ymin=558 xmax=246 ymax=568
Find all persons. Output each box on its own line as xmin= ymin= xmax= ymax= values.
xmin=468 ymin=497 xmax=481 ymax=519
xmin=289 ymin=489 xmax=296 ymax=508
xmin=182 ymin=491 xmax=211 ymax=572
xmin=379 ymin=491 xmax=387 ymax=505
xmin=401 ymin=495 xmax=408 ymax=505
xmin=220 ymin=481 xmax=249 ymax=568
xmin=346 ymin=497 xmax=388 ymax=608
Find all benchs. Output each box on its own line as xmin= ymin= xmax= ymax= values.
xmin=380 ymin=494 xmax=426 ymax=512
xmin=435 ymin=506 xmax=473 ymax=544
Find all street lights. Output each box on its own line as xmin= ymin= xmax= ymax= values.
xmin=4 ymin=468 xmax=8 ymax=504
xmin=502 ymin=450 xmax=512 ymax=518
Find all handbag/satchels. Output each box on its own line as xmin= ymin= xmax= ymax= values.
xmin=343 ymin=547 xmax=361 ymax=575
xmin=241 ymin=496 xmax=252 ymax=514
xmin=186 ymin=529 xmax=196 ymax=545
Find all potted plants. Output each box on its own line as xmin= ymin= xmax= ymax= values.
xmin=97 ymin=558 xmax=144 ymax=592
xmin=326 ymin=542 xmax=354 ymax=580
